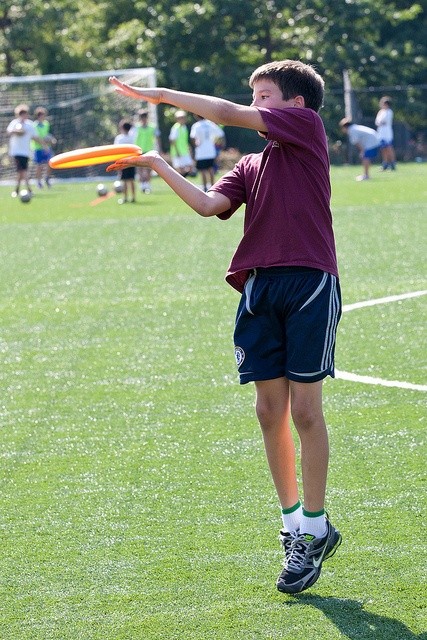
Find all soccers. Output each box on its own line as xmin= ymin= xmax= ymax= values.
xmin=19 ymin=189 xmax=31 ymax=202
xmin=112 ymin=180 xmax=123 ymax=192
xmin=95 ymin=183 xmax=106 ymax=196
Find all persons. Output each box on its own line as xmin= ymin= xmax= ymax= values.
xmin=107 ymin=59 xmax=342 ymax=594
xmin=339 ymin=117 xmax=381 ymax=180
xmin=7 ymin=104 xmax=50 ymax=196
xmin=169 ymin=110 xmax=193 ymax=178
xmin=133 ymin=110 xmax=155 ymax=193
xmin=114 ymin=119 xmax=134 ymax=204
xmin=375 ymin=95 xmax=396 ymax=170
xmin=190 ymin=112 xmax=225 ymax=192
xmin=29 ymin=107 xmax=56 ymax=189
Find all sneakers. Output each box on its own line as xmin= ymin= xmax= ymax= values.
xmin=275 ymin=516 xmax=342 ymax=593
xmin=279 ymin=526 xmax=300 ymax=566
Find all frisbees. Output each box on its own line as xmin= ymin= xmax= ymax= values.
xmin=48 ymin=144 xmax=143 ymax=170
